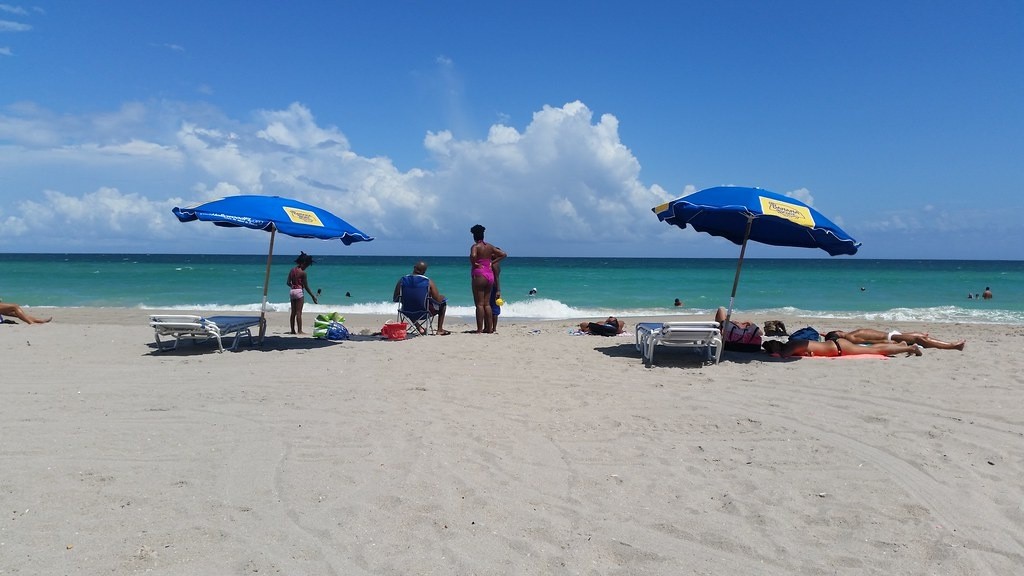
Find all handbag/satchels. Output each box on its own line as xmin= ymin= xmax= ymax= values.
xmin=324 ymin=321 xmax=349 ymax=341
xmin=312 ymin=311 xmax=346 ymax=338
xmin=720 ymin=320 xmax=762 ymax=353
xmin=764 ymin=320 xmax=787 ymax=336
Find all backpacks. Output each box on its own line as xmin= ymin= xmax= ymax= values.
xmin=788 ymin=326 xmax=820 ymax=342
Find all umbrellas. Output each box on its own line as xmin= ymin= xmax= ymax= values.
xmin=172 ymin=195 xmax=376 ymax=346
xmin=652 ymin=186 xmax=862 ymax=362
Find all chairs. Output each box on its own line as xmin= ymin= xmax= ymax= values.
xmin=397 ymin=275 xmax=447 ymax=335
xmin=148 ymin=315 xmax=267 ymax=353
xmin=636 ymin=320 xmax=723 ymax=367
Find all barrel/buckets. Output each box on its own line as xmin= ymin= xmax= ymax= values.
xmin=495 ymin=297 xmax=503 ymax=306
xmin=385 ymin=319 xmax=408 ymax=340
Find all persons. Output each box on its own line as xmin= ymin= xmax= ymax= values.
xmin=317 ymin=289 xmax=353 ymax=298
xmin=0 ymin=302 xmax=53 ymax=324
xmin=714 ymin=285 xmax=992 ymax=357
xmin=287 ymin=251 xmax=318 ymax=335
xmin=393 ymin=261 xmax=451 ymax=335
xmin=470 ymin=224 xmax=626 ymax=337
xmin=674 ymin=298 xmax=682 ymax=308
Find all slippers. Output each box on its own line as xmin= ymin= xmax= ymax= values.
xmin=436 ymin=330 xmax=451 ymax=335
xmin=420 ymin=329 xmax=426 ymax=335
